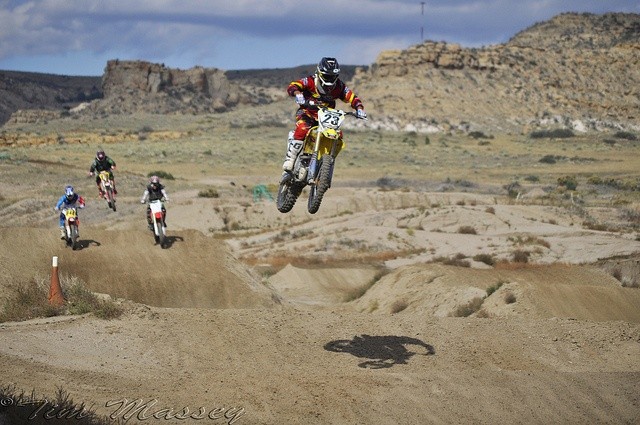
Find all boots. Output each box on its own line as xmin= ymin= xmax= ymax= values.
xmin=283 ymin=139 xmax=304 ymax=171
xmin=60 ymin=229 xmax=66 ymax=240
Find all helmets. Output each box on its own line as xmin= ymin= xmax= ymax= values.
xmin=316 ymin=56 xmax=341 ymax=88
xmin=64 ymin=185 xmax=75 ymax=201
xmin=150 ymin=176 xmax=159 ymax=191
xmin=96 ymin=150 xmax=106 ymax=162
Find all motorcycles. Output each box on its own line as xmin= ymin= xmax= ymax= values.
xmin=143 ymin=198 xmax=167 ymax=248
xmin=55 ymin=205 xmax=84 ymax=250
xmin=277 ymin=98 xmax=368 ymax=214
xmin=93 ymin=169 xmax=117 ymax=211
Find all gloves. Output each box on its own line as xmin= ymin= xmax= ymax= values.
xmin=80 ymin=204 xmax=85 ymax=209
xmin=356 ymin=109 xmax=367 ymax=119
xmin=295 ymin=93 xmax=306 ymax=105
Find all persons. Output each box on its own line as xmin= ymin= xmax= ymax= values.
xmin=283 ymin=57 xmax=367 ymax=172
xmin=140 ymin=175 xmax=169 ymax=228
xmin=54 ymin=186 xmax=85 ymax=240
xmin=89 ymin=149 xmax=117 ymax=198
